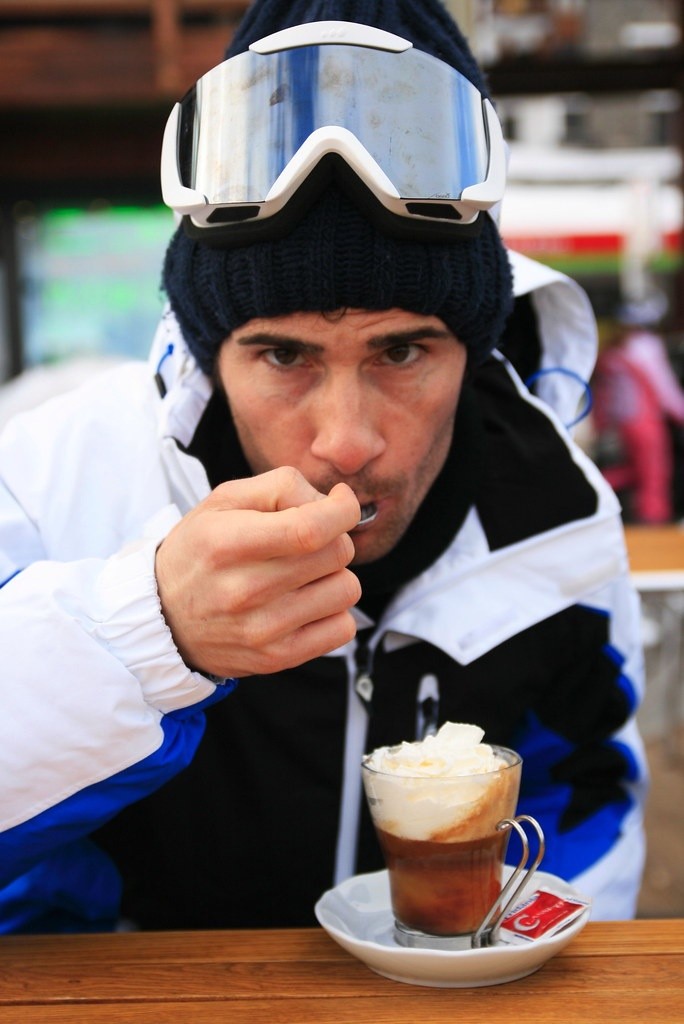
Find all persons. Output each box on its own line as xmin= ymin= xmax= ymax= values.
xmin=587 ymin=286 xmax=684 ymax=523
xmin=0 ymin=0 xmax=648 ymax=936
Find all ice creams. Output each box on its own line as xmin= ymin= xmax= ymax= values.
xmin=365 ymin=721 xmax=516 ymax=843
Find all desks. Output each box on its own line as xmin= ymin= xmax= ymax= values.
xmin=0 ymin=917 xmax=684 ymax=1024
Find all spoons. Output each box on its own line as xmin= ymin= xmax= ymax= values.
xmin=359 ymin=504 xmax=378 ymax=524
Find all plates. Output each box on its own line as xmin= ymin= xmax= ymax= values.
xmin=314 ymin=866 xmax=594 ymax=991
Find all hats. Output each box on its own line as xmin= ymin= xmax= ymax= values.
xmin=163 ymin=0 xmax=513 ymax=362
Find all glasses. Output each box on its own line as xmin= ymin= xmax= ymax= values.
xmin=160 ymin=22 xmax=506 ymax=226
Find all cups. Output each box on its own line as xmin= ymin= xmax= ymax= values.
xmin=361 ymin=744 xmax=546 ymax=950
